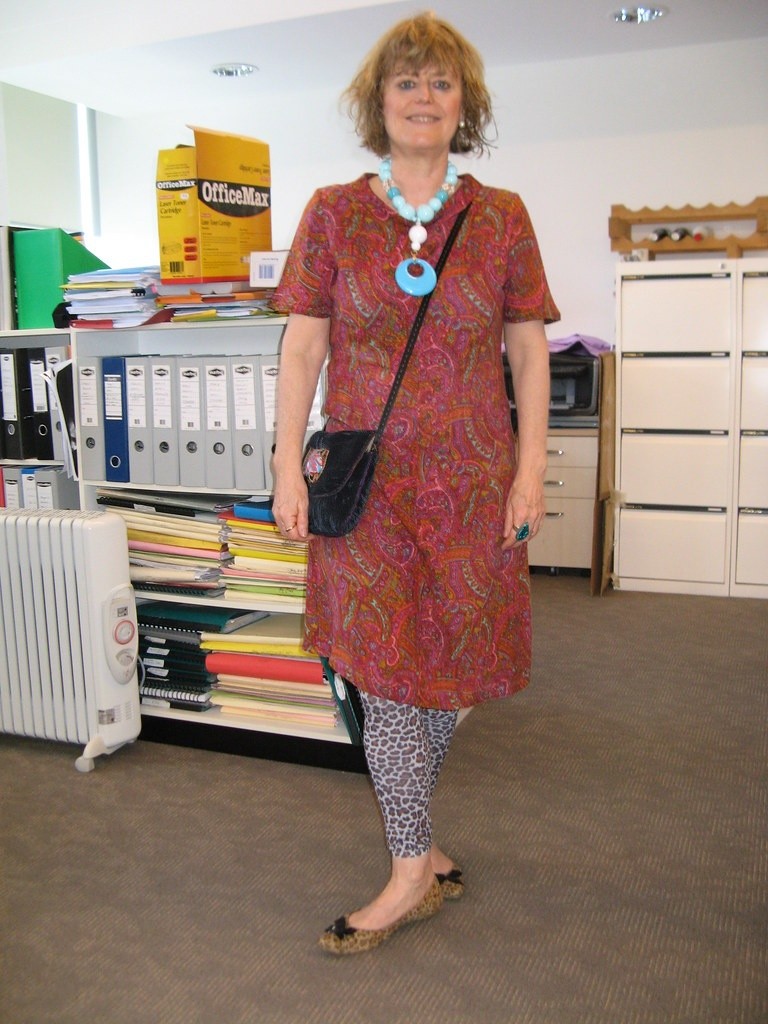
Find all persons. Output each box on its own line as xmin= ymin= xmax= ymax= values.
xmin=270 ymin=14 xmax=551 ymax=959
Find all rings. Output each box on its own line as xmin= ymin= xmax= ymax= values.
xmin=286 ymin=523 xmax=297 ymax=532
xmin=512 ymin=521 xmax=529 ymax=540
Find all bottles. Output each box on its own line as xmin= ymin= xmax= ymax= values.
xmin=649 ymin=228 xmax=671 ymax=242
xmin=671 ymin=228 xmax=693 ymax=241
xmin=693 ymin=225 xmax=714 ymax=242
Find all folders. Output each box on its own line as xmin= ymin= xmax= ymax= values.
xmin=0 ymin=344 xmax=330 ymax=512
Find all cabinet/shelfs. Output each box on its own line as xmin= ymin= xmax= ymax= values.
xmin=515 ymin=426 xmax=602 ymax=598
xmin=0 ymin=314 xmax=371 ymax=778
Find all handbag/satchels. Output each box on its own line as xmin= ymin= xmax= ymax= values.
xmin=301 ymin=430 xmax=378 ymax=537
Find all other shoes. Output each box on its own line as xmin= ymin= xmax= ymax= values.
xmin=431 ymin=843 xmax=465 ymax=900
xmin=318 ymin=874 xmax=444 ymax=955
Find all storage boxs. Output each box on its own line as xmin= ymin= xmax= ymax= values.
xmin=155 ymin=122 xmax=272 ymax=287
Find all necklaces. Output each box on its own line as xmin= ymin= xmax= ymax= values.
xmin=379 ymin=155 xmax=457 ymax=297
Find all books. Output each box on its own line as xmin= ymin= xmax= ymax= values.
xmin=95 ymin=489 xmax=342 ymax=728
xmin=58 ymin=265 xmax=276 ymax=331
